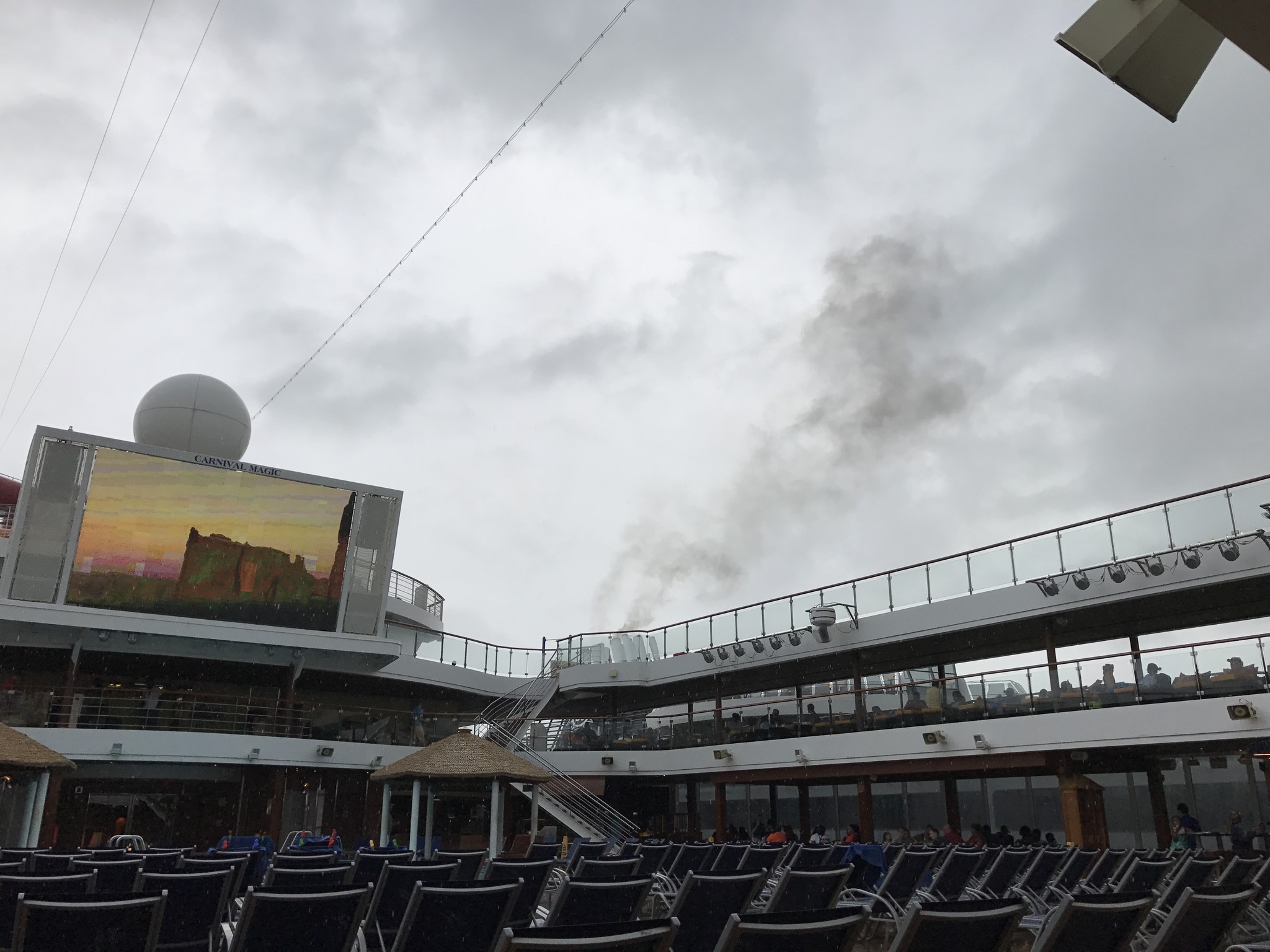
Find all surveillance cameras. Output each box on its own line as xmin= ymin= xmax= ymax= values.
xmin=317 ymin=744 xmax=334 ymax=756
xmin=712 ymin=749 xmax=731 ymax=759
xmin=922 ymin=731 xmax=946 ymax=744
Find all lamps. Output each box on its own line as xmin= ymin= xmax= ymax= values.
xmin=1227 ymin=697 xmax=1257 ymax=721
xmin=768 ymin=634 xmax=782 ymax=651
xmin=701 ymin=649 xmax=714 ymax=663
xmin=714 ymin=749 xmax=731 ymax=759
xmin=628 ymin=760 xmax=637 ymax=771
xmin=1217 ymin=536 xmax=1240 ymax=561
xmin=923 ymin=731 xmax=946 ymax=746
xmin=1041 ymin=574 xmax=1061 ymax=597
xmin=370 ymin=756 xmax=383 ymax=768
xmin=1144 ymin=552 xmax=1165 ymax=576
xmin=795 ymin=748 xmax=808 ymax=763
xmin=716 ymin=644 xmax=729 ymax=661
xmin=1107 ymin=561 xmax=1127 ymax=583
xmin=732 ymin=642 xmax=745 ymax=657
xmin=1180 ymin=544 xmax=1201 ymax=570
xmin=752 ymin=638 xmax=765 ymax=653
xmin=973 ymin=734 xmax=990 ymax=750
xmin=601 ymin=755 xmax=615 ymax=766
xmin=316 ymin=744 xmax=334 ymax=757
xmin=1071 ymin=567 xmax=1091 ymax=589
xmin=788 ymin=631 xmax=801 ymax=647
xmin=248 ymin=747 xmax=260 ymax=760
xmin=111 ymin=742 xmax=123 ymax=757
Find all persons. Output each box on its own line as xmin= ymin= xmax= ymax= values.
xmin=638 ymin=802 xmax=1252 ymax=850
xmin=552 ymin=658 xmax=1265 ymax=751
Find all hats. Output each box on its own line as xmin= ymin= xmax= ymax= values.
xmin=912 ymin=692 xmax=920 ymax=695
xmin=1227 ymin=657 xmax=1243 ymax=664
xmin=1003 ymin=687 xmax=1014 ymax=693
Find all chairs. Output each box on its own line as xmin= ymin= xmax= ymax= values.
xmin=0 ymin=836 xmax=1270 ymax=952
xmin=566 ymin=664 xmax=1261 ymax=749
